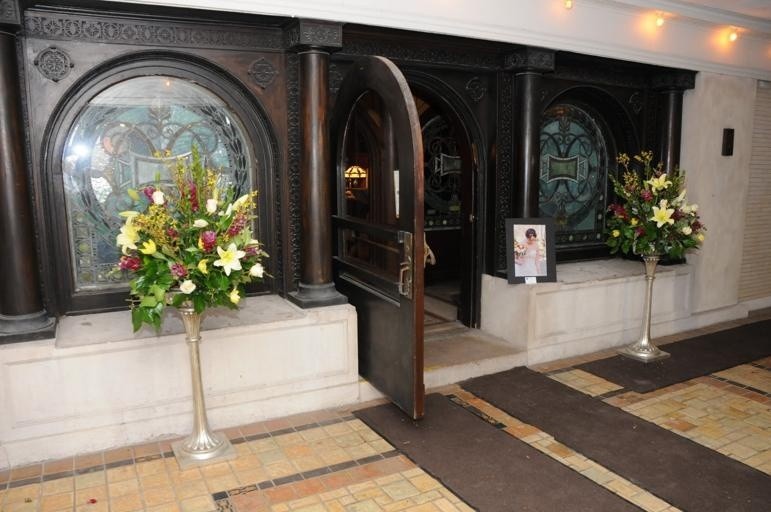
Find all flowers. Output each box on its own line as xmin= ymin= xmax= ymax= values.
xmin=117 ymin=150 xmax=275 ymax=335
xmin=595 ymin=147 xmax=709 ymax=267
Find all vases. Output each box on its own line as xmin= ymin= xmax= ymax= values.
xmin=157 ymin=286 xmax=244 ymax=475
xmin=617 ymin=245 xmax=675 ymax=368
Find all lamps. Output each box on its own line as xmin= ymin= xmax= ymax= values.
xmin=341 ymin=158 xmax=375 ymax=193
xmin=559 ymin=0 xmax=742 ymax=42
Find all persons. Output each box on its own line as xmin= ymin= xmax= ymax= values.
xmin=515 ymin=228 xmax=543 ymax=276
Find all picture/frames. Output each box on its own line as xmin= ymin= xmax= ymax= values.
xmin=503 ymin=215 xmax=560 ymax=286
